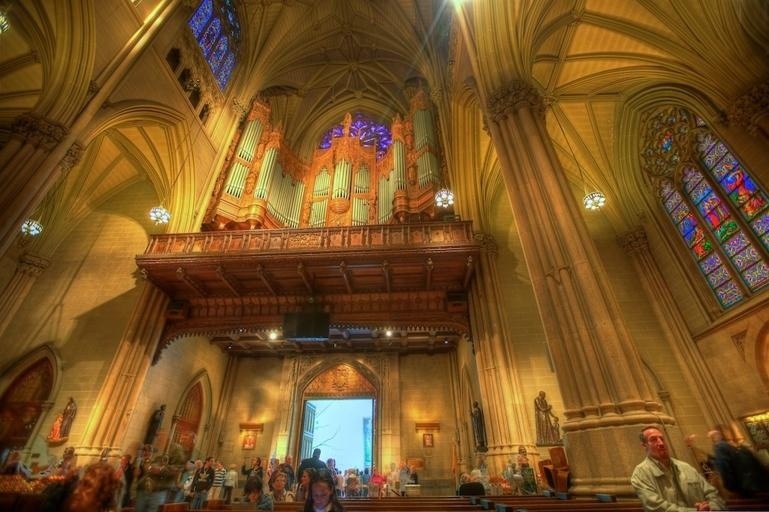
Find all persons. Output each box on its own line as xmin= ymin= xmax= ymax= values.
xmin=534 ymin=390 xmax=556 ymax=444
xmin=456 ymin=446 xmax=532 ymax=498
xmin=1 ymin=443 xmax=421 ymax=512
xmin=468 ymin=400 xmax=485 ymax=448
xmin=682 ymin=166 xmax=765 ymax=259
xmin=552 ymin=417 xmax=561 ymax=440
xmin=630 ymin=425 xmax=730 ymax=511
xmin=708 ymin=430 xmax=768 ymax=500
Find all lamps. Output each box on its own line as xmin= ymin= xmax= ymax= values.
xmin=147 ymin=112 xmax=213 ymax=225
xmin=17 ymin=134 xmax=101 ymax=241
xmin=434 ymin=174 xmax=456 ymax=209
xmin=548 ymin=104 xmax=606 ymax=211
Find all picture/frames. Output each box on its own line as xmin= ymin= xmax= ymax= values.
xmin=241 ymin=434 xmax=255 ymax=449
xmin=423 ymin=433 xmax=434 ymax=448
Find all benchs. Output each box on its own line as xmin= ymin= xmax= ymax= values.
xmin=159 ymin=490 xmax=767 ymax=512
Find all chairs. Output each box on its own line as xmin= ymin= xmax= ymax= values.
xmin=459 ymin=481 xmax=486 ymax=496
